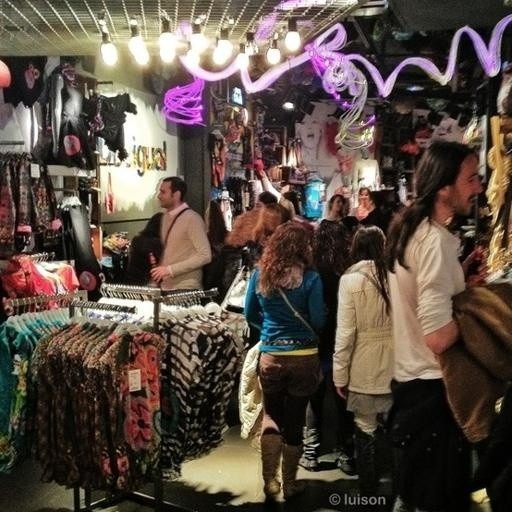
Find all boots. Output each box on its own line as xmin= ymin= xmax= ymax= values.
xmin=299 ymin=427 xmax=319 ymax=472
xmin=281 ymin=443 xmax=306 ymax=500
xmin=337 ymin=432 xmax=357 ymax=474
xmin=374 ymin=413 xmax=394 ymax=496
xmin=357 ymin=424 xmax=380 ymax=511
xmin=259 ymin=433 xmax=281 ymax=497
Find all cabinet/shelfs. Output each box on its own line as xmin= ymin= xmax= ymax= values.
xmin=378 ymin=103 xmax=420 ymax=210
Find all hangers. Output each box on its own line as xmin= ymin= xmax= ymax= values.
xmin=55 ymin=186 xmax=90 ymax=211
xmin=219 ymin=184 xmax=232 ymax=203
xmin=2 ymin=276 xmax=229 ymax=339
xmin=0 ymin=139 xmax=39 ymax=165
xmin=307 ymin=171 xmax=323 ymax=182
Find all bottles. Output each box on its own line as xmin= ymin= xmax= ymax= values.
xmin=105 ymin=172 xmax=115 ymax=216
xmin=148 ymin=252 xmax=157 ymax=268
xmin=467 ymin=240 xmax=484 ymax=278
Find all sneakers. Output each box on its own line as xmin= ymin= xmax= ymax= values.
xmin=251 ymin=432 xmax=261 ymax=453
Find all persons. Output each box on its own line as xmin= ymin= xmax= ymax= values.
xmin=130 ymin=141 xmax=512 ymax=512
xmin=296 ymin=101 xmax=338 ymax=162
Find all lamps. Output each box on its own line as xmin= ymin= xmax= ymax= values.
xmin=87 ymin=5 xmax=307 ymax=69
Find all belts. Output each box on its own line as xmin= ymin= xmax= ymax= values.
xmin=264 ymin=338 xmax=317 ymax=346
